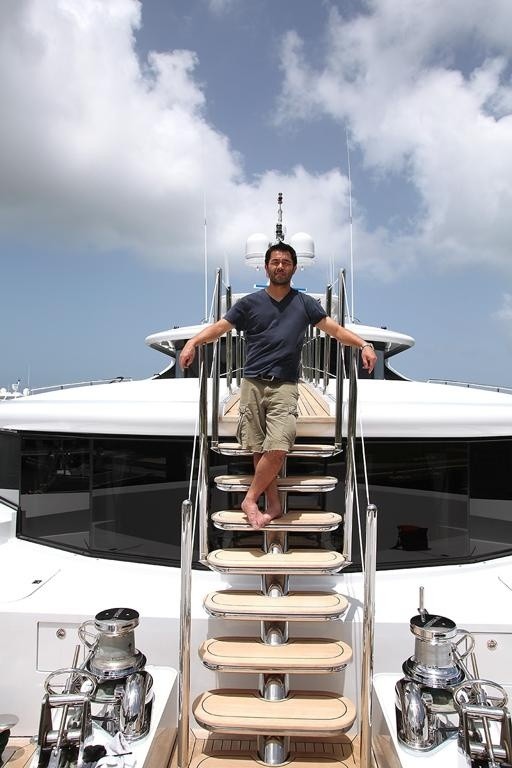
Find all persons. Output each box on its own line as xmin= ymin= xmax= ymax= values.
xmin=178 ymin=241 xmax=378 ymax=531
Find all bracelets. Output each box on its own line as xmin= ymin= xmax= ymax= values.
xmin=360 ymin=342 xmax=375 ymax=351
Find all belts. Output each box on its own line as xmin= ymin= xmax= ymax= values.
xmin=251 ymin=372 xmax=279 ymax=383
